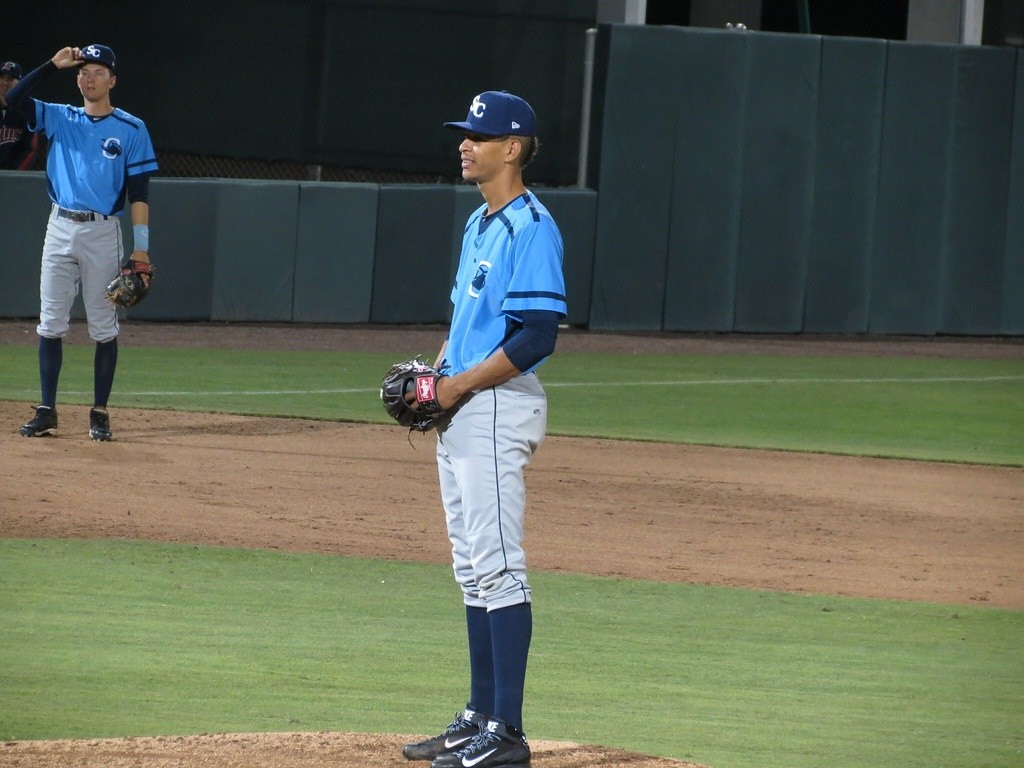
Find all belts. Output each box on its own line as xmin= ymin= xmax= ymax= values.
xmin=57 ymin=207 xmax=108 ymax=222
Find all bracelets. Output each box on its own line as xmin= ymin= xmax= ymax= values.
xmin=133 ymin=224 xmax=149 ymax=252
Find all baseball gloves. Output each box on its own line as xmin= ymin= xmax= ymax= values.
xmin=105 ymin=260 xmax=156 ymax=308
xmin=378 ymin=359 xmax=451 ymax=433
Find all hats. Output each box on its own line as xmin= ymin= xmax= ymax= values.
xmin=0 ymin=62 xmax=22 ymax=80
xmin=444 ymin=90 xmax=537 ymax=138
xmin=77 ymin=44 xmax=116 ymax=69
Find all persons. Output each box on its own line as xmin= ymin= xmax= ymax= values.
xmin=378 ymin=89 xmax=568 ymax=768
xmin=5 ymin=45 xmax=159 ymax=442
xmin=0 ymin=61 xmax=39 ymax=171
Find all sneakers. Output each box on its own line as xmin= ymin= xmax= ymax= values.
xmin=89 ymin=408 xmax=112 ymax=441
xmin=19 ymin=405 xmax=57 ymax=436
xmin=430 ymin=716 xmax=531 ymax=768
xmin=403 ymin=709 xmax=484 ymax=760
